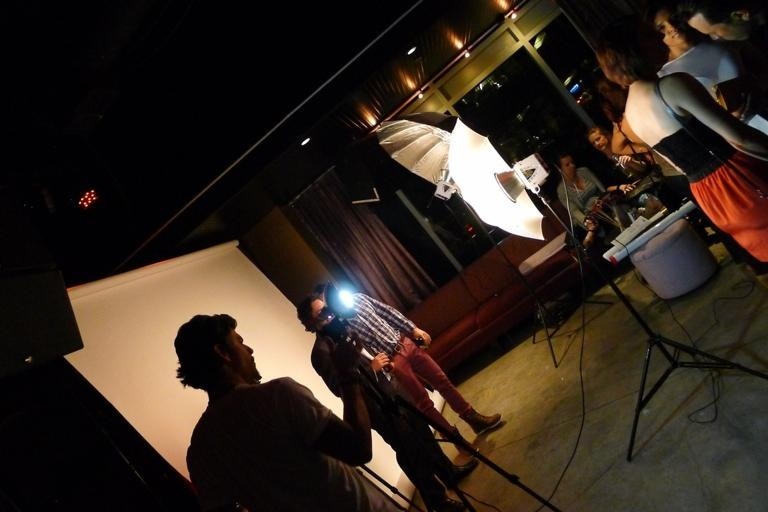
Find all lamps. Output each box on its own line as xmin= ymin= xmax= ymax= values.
xmin=376 ymin=111 xmax=768 ymax=461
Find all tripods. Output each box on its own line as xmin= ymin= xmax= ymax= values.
xmin=454 ymin=192 xmax=614 ymax=369
xmin=389 ymin=397 xmax=563 ymax=512
xmin=540 ymin=196 xmax=768 ymax=462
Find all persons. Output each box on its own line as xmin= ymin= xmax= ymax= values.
xmin=293 ymin=293 xmax=482 ymax=512
xmin=555 ymin=3 xmax=768 ymax=291
xmin=174 ymin=313 xmax=402 ymax=510
xmin=308 ymin=281 xmax=502 ymax=456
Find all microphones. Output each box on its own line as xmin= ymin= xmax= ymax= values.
xmin=612 ymin=155 xmax=624 ymax=168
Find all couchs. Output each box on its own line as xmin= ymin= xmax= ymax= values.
xmin=402 ymin=208 xmax=587 ymax=384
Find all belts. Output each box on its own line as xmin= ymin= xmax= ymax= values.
xmin=388 ymin=334 xmax=405 ymax=360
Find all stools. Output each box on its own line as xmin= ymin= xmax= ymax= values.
xmin=626 ymin=217 xmax=721 ymax=303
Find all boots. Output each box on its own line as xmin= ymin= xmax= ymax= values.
xmin=451 ymin=427 xmax=480 ymax=459
xmin=460 ymin=406 xmax=503 ymax=437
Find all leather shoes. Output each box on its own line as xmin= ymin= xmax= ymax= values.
xmin=580 ymin=248 xmax=594 ymax=265
xmin=432 ymin=495 xmax=466 ymax=511
xmin=445 ymin=459 xmax=479 ymax=487
